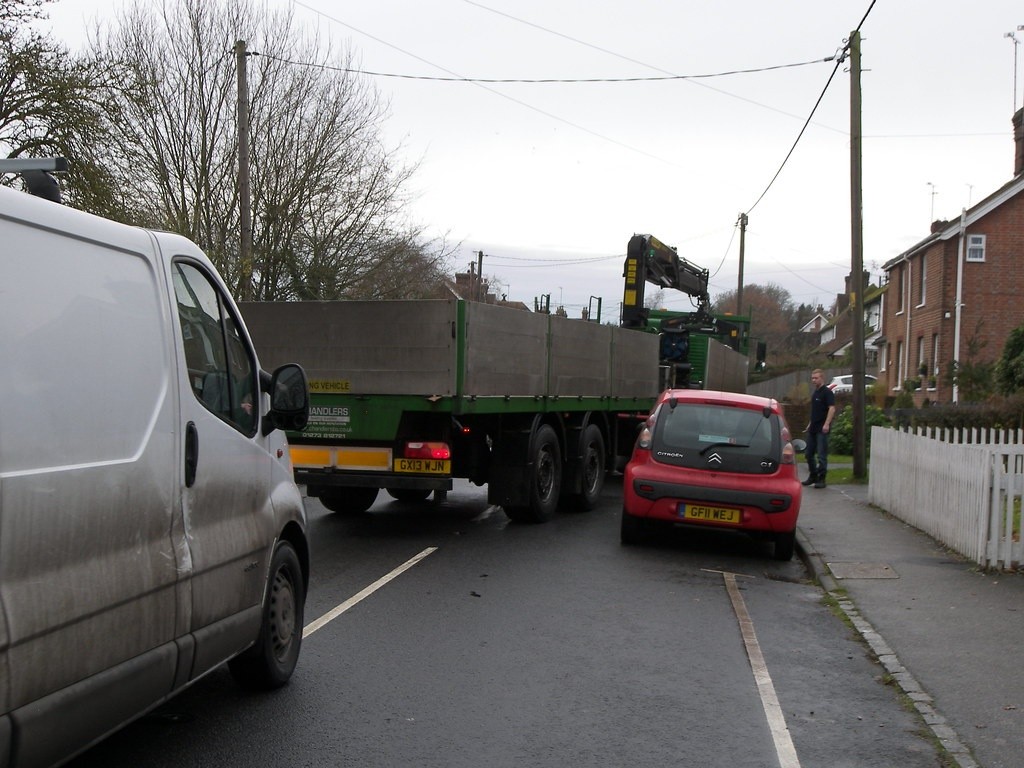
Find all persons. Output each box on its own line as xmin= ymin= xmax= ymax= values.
xmin=801 ymin=369 xmax=835 ymax=489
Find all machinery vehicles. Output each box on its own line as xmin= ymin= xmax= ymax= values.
xmin=618 ymin=232 xmax=740 ymax=387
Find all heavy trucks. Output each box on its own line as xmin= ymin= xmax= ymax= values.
xmin=221 ymin=302 xmax=766 ymax=526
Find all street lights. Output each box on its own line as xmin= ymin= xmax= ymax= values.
xmin=1004 ymin=23 xmax=1023 ymax=113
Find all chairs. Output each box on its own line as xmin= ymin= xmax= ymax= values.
xmin=732 ymin=413 xmax=771 ymax=444
xmin=672 ymin=407 xmax=700 ymax=432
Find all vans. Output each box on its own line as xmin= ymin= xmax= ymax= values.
xmin=1 ymin=186 xmax=310 ymax=768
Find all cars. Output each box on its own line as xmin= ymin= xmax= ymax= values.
xmin=619 ymin=388 xmax=809 ymax=562
xmin=827 ymin=374 xmax=879 ymax=395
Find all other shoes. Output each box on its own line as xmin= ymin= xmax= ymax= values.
xmin=815 ymin=479 xmax=826 ymax=489
xmin=801 ymin=476 xmax=817 ymax=485
